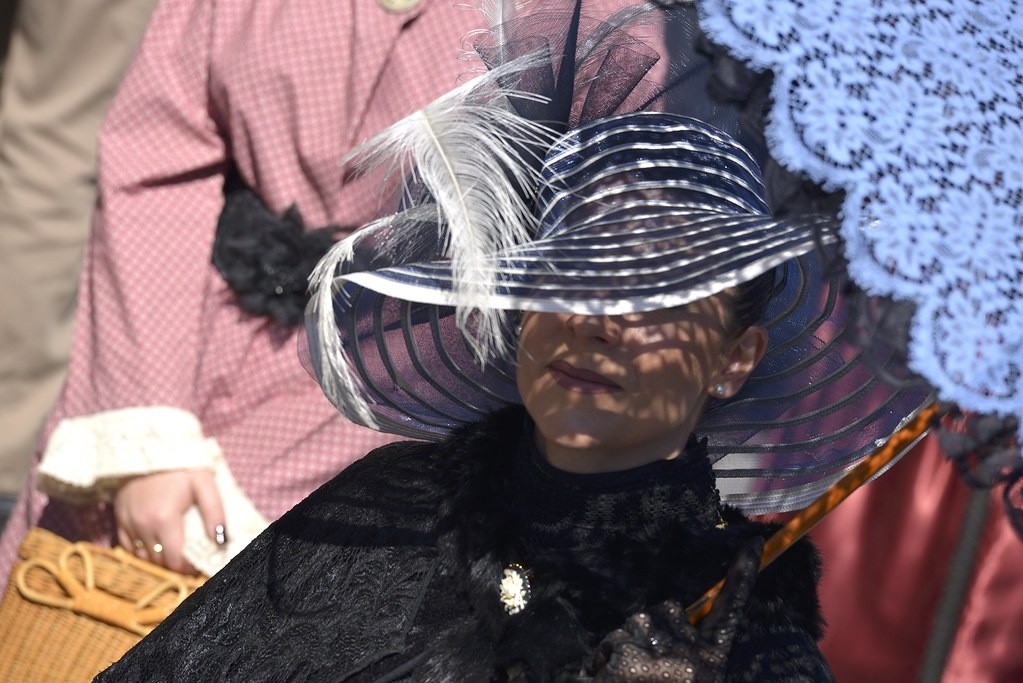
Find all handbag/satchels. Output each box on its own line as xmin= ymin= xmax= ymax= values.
xmin=0 ymin=528 xmax=207 ymax=683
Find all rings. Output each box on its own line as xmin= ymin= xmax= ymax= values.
xmin=679 ymin=626 xmax=698 ymax=640
xmin=148 ymin=546 xmax=162 ymax=554
xmin=134 ymin=542 xmax=144 ymax=549
xmin=648 ymin=630 xmax=672 ymax=653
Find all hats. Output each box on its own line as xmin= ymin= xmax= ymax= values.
xmin=297 ymin=1 xmax=940 ymax=515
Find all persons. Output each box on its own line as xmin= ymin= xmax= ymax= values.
xmin=0 ymin=0 xmax=741 ymax=683
xmin=91 ymin=110 xmax=943 ymax=682
